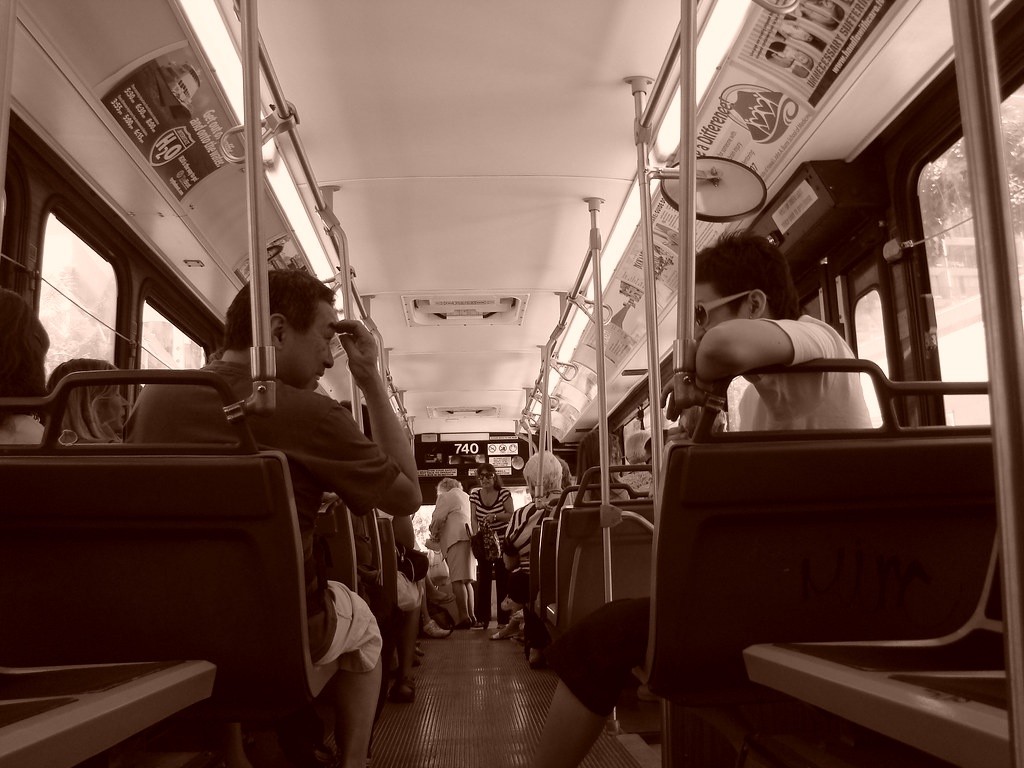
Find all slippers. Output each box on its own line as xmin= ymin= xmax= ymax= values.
xmin=489 ymin=629 xmax=521 ymax=640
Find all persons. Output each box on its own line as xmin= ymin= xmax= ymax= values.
xmin=124 ymin=270 xmax=422 ymax=768
xmin=469 ymin=463 xmax=514 ymax=629
xmin=0 ymin=287 xmax=51 ymax=443
xmin=489 ymin=406 xmax=728 ymax=670
xmin=46 ymin=358 xmax=129 ymax=444
xmin=526 ymin=236 xmax=875 ymax=768
xmin=429 ymin=478 xmax=480 ymax=629
xmin=334 ymin=401 xmax=455 ymax=700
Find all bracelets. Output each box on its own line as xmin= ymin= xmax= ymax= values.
xmin=493 ymin=514 xmax=496 ymax=521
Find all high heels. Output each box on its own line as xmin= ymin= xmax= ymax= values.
xmin=469 ymin=616 xmax=475 ymax=627
xmin=496 ymin=623 xmax=509 ymax=629
xmin=473 ymin=620 xmax=489 ymax=628
xmin=454 ymin=618 xmax=472 ymax=630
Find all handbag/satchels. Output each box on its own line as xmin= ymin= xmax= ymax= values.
xmin=396 ymin=543 xmax=430 ymax=583
xmin=425 ymin=538 xmax=441 ymax=553
xmin=465 ymin=519 xmax=498 ymax=567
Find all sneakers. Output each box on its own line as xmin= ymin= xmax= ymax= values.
xmin=422 ymin=620 xmax=453 ymax=639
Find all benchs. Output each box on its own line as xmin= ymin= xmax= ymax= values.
xmin=0 ymin=369 xmax=431 ymax=768
xmin=507 ymin=344 xmax=1023 ymax=768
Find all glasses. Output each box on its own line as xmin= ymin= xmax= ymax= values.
xmin=693 ymin=289 xmax=767 ymax=328
xmin=478 ymin=472 xmax=495 ymax=480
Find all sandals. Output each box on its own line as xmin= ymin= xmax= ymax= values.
xmin=389 ymin=676 xmax=414 ymax=703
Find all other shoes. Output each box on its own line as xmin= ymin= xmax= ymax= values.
xmin=529 ymin=649 xmax=544 ymax=669
xmin=412 ymin=655 xmax=420 ymax=667
xmin=413 ymin=644 xmax=425 ymax=656
